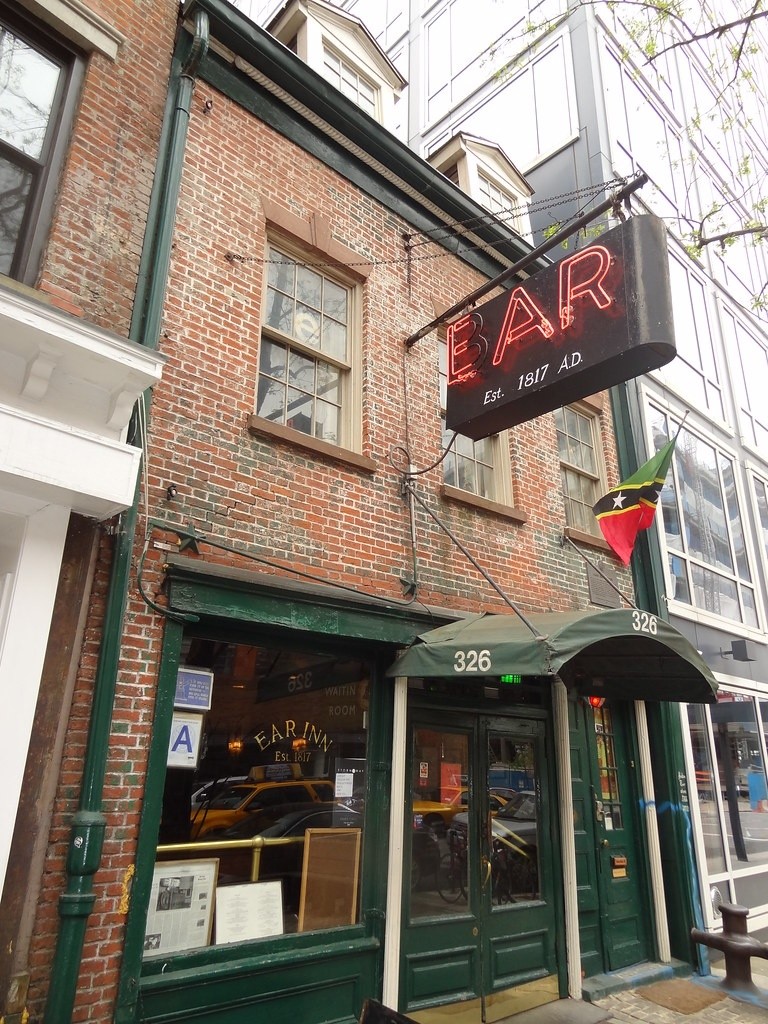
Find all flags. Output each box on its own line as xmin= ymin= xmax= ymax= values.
xmin=591 ymin=436 xmax=677 ymax=565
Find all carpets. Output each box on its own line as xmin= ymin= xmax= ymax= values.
xmin=491 ymin=998 xmax=613 ymax=1024
xmin=636 ymin=977 xmax=729 ymax=1015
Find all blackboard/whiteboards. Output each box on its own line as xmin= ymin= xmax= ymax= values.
xmin=296 ymin=827 xmax=362 ymax=930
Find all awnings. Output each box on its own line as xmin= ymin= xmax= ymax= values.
xmin=382 ymin=608 xmax=722 ymax=704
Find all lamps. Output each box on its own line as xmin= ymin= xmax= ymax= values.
xmin=292 ymin=732 xmax=306 ymax=754
xmin=227 ymin=726 xmax=244 ymax=757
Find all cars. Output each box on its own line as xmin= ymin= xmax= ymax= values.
xmin=192 ymin=774 xmax=539 ymax=904
